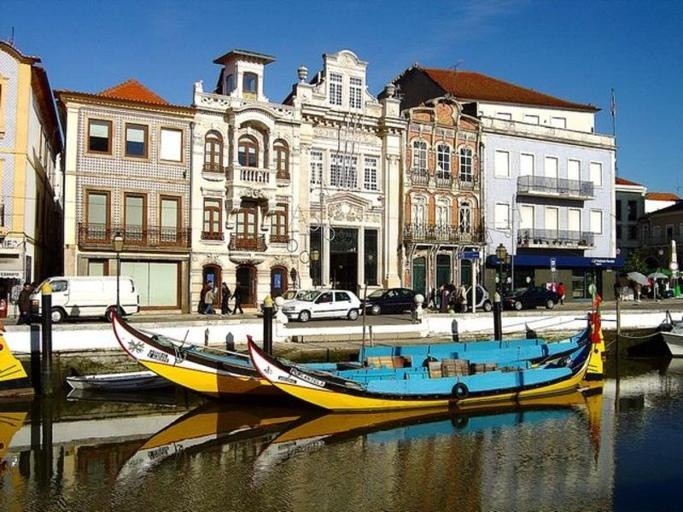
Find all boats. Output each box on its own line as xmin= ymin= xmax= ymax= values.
xmin=66 ymin=294 xmax=606 ymax=412
xmin=114 ymin=380 xmax=605 ymax=483
xmin=659 ymin=321 xmax=683 ymax=356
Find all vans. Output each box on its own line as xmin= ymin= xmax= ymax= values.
xmin=28 ymin=275 xmax=140 ymax=323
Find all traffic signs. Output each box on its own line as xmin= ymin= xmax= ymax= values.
xmin=458 ymin=252 xmax=480 ymax=259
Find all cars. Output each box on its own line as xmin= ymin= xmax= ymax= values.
xmin=504 ymin=286 xmax=560 ymax=310
xmin=253 ymin=288 xmax=428 ymax=322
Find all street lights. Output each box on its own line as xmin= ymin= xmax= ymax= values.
xmin=658 ymin=249 xmax=664 ymax=274
xmin=113 ymin=231 xmax=123 ymax=315
xmin=310 ymin=249 xmax=320 ymax=289
xmin=496 ymin=243 xmax=506 ymax=311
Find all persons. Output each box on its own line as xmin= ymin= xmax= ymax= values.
xmin=198 ymin=280 xmax=243 ymax=316
xmin=541 ymin=279 xmax=566 ymax=310
xmin=427 ymin=281 xmax=463 ymax=312
xmin=16 ymin=283 xmax=33 ymax=325
xmin=629 ymin=278 xmax=672 ymax=299
